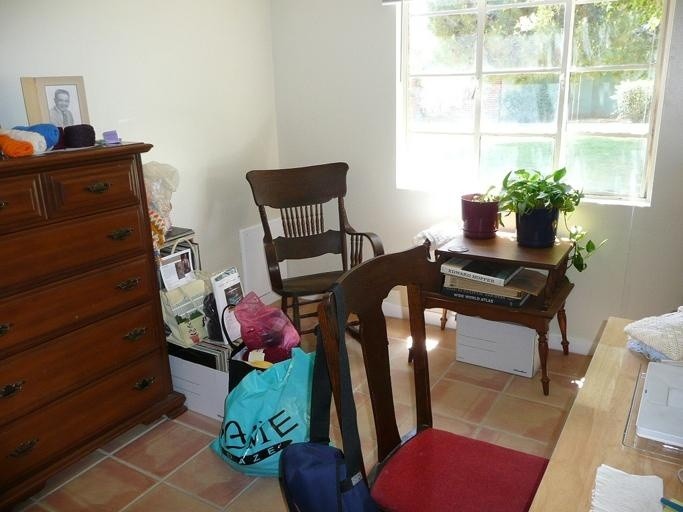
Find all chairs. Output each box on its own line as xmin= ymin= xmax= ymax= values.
xmin=245 ymin=161 xmax=388 ymax=348
xmin=311 ymin=239 xmax=550 ymax=512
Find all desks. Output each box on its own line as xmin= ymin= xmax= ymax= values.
xmin=526 ymin=315 xmax=683 ymax=512
xmin=407 ymin=227 xmax=575 ymax=399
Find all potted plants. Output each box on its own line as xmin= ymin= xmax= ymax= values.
xmin=461 ymin=185 xmax=504 ymax=239
xmin=496 ymin=167 xmax=609 ymax=273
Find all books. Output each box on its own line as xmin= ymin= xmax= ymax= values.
xmin=439 ymin=254 xmax=546 ymax=308
xmin=153 ymin=222 xmax=257 ymax=375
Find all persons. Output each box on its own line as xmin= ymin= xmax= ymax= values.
xmin=49 ymin=89 xmax=74 ymax=128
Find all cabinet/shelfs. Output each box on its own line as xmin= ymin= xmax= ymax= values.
xmin=0 ymin=140 xmax=186 ymax=511
xmin=151 ymin=226 xmax=207 ymax=341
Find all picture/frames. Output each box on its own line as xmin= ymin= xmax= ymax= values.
xmin=17 ymin=74 xmax=91 ymax=132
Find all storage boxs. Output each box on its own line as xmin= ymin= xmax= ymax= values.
xmin=453 ymin=313 xmax=551 ymax=379
xmin=165 ymin=351 xmax=228 ymax=424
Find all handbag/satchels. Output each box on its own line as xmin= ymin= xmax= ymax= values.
xmin=157 ymin=235 xmax=215 ymax=349
xmin=280 ymin=281 xmax=379 ymax=511
xmin=221 ymin=300 xmax=301 ymax=394
